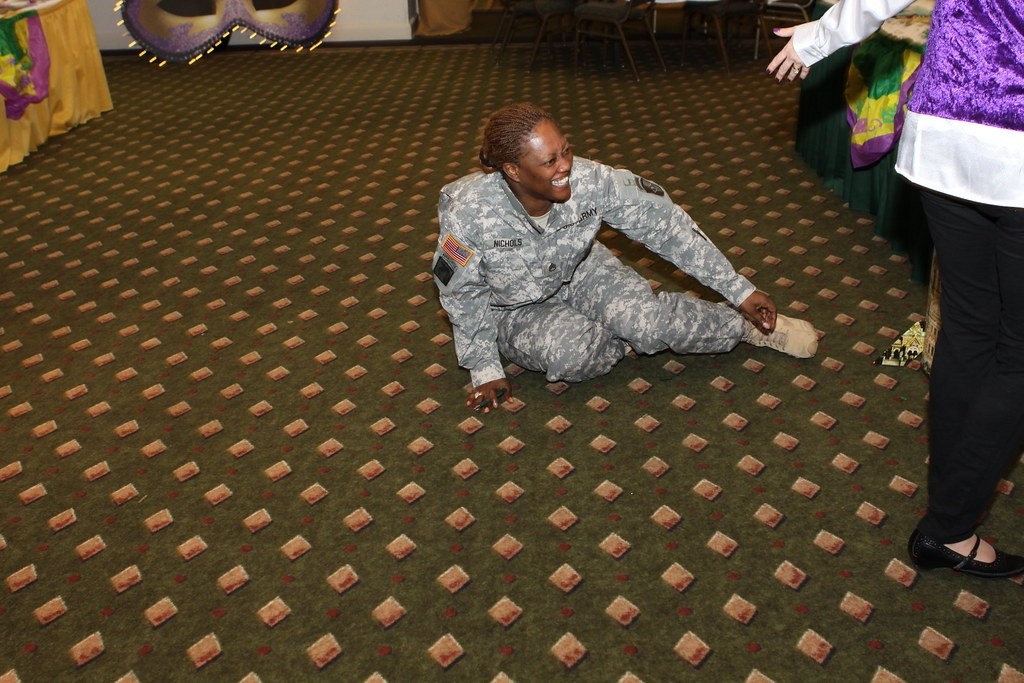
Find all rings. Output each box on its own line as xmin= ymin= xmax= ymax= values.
xmin=474 ymin=391 xmax=482 ymax=399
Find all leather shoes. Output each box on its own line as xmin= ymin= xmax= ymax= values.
xmin=907 ymin=526 xmax=1024 ymax=576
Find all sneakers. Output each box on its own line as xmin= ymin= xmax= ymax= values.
xmin=623 ymin=341 xmax=632 ymax=356
xmin=740 ymin=312 xmax=818 ymax=359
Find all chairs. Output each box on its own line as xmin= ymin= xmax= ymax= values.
xmin=488 ymin=0 xmax=815 ymax=84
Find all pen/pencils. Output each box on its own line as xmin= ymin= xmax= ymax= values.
xmin=474 ymin=388 xmax=507 ymax=412
xmin=692 ymin=228 xmax=707 ymax=242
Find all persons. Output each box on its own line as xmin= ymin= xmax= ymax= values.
xmin=431 ymin=105 xmax=819 ymax=412
xmin=766 ymin=0 xmax=1023 ymax=579
